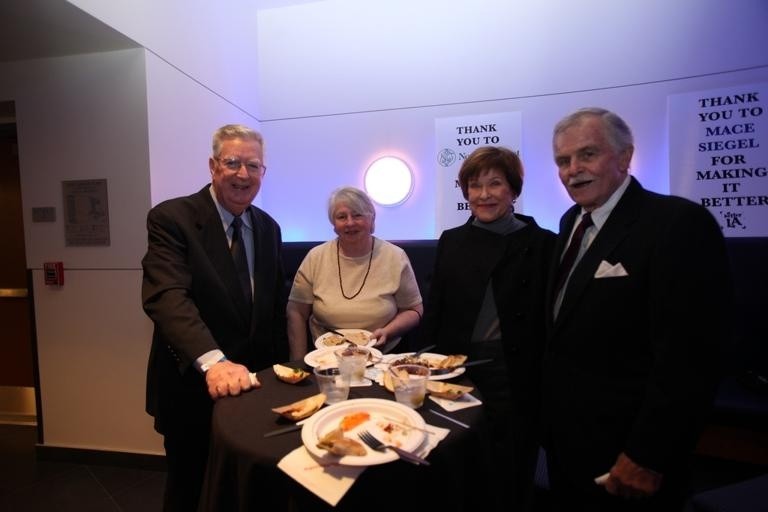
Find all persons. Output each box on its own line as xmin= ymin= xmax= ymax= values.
xmin=525 ymin=108 xmax=731 ymax=512
xmin=412 ymin=146 xmax=558 ymax=512
xmin=141 ymin=122 xmax=290 ymax=512
xmin=286 ymin=186 xmax=426 ymax=363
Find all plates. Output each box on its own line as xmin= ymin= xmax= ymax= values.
xmin=315 ymin=328 xmax=376 ymax=349
xmin=302 ymin=397 xmax=424 ymax=468
xmin=304 ymin=346 xmax=384 ymax=372
xmin=385 ymin=352 xmax=468 ymax=383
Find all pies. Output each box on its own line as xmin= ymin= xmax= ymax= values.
xmin=324 ymin=333 xmax=369 ymax=347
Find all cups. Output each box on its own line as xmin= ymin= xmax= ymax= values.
xmin=314 ymin=361 xmax=353 ymax=405
xmin=332 ymin=349 xmax=372 ymax=384
xmin=390 ymin=364 xmax=430 ymax=409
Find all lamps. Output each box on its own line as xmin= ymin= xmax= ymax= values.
xmin=363 ymin=154 xmax=415 ymax=207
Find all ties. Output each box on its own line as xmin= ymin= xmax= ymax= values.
xmin=231 ymin=217 xmax=253 ymax=320
xmin=552 ymin=213 xmax=594 ymax=308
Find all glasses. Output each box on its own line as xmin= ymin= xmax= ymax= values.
xmin=215 ymin=156 xmax=267 ymax=178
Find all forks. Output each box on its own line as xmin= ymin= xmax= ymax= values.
xmin=356 ymin=429 xmax=430 ymax=470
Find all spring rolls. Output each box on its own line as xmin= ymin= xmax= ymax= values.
xmin=317 ymin=439 xmax=366 ymax=457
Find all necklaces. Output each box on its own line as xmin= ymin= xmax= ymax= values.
xmin=337 ymin=235 xmax=375 ymax=299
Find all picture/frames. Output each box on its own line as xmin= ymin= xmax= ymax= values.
xmin=60 ymin=177 xmax=109 ymax=248
